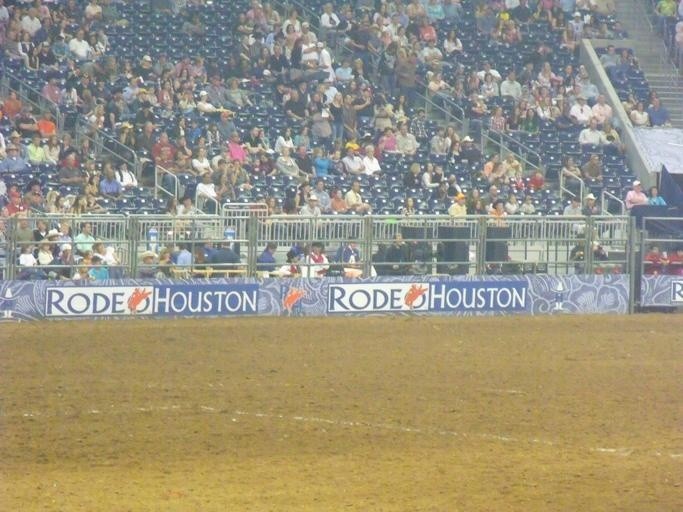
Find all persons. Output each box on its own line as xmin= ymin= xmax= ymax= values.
xmin=1 ymin=1 xmax=683 ymax=277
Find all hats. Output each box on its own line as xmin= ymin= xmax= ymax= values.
xmin=45 ymin=229 xmax=64 ymax=239
xmin=460 ymin=135 xmax=474 ymax=144
xmin=8 ymin=131 xmax=22 ymax=139
xmin=41 ymin=41 xmax=49 ymax=47
xmin=93 ymin=238 xmax=102 ymax=250
xmin=142 ymin=54 xmax=150 ymax=62
xmin=456 ymin=193 xmax=465 ymax=201
xmin=199 ymin=90 xmax=208 ymax=97
xmin=584 ymin=193 xmax=598 ymax=201
xmin=139 ymin=250 xmax=158 ymax=261
xmin=298 ymin=181 xmax=311 ymax=191
xmin=5 ymin=144 xmax=18 ymax=152
xmin=315 ymin=42 xmax=323 ymax=47
xmin=632 ymin=180 xmax=641 ymax=187
xmin=39 ymin=239 xmax=48 ymax=248
xmin=306 ymin=194 xmax=320 ymax=202
